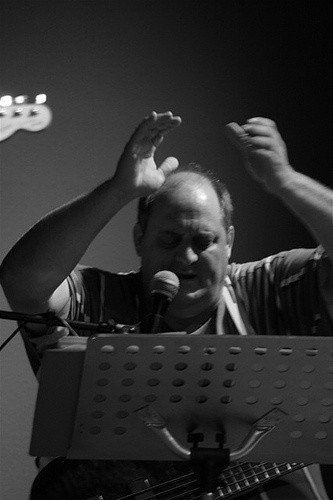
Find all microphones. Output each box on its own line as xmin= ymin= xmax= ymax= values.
xmin=142 ymin=271 xmax=180 ymax=335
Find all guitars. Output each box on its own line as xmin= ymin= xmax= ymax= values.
xmin=28 ymin=456 xmax=312 ymax=500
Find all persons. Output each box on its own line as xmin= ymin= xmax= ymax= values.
xmin=1 ymin=112 xmax=332 ymax=500
